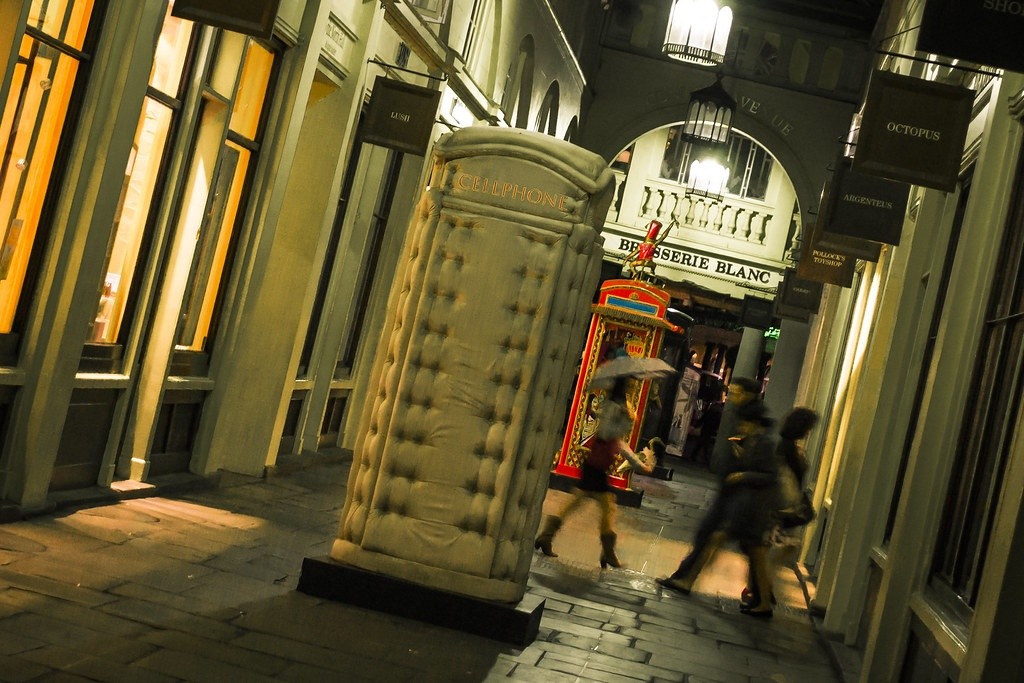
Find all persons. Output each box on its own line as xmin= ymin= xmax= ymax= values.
xmin=689 ymin=402 xmax=725 ymax=462
xmin=534 ymin=372 xmax=652 ymax=570
xmin=652 ymin=398 xmax=776 ymax=619
xmin=741 ymin=406 xmax=819 ymax=607
xmin=623 ymin=217 xmax=679 ymax=279
xmin=670 ymin=376 xmax=777 ymax=610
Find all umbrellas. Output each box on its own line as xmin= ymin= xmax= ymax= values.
xmin=588 ymin=355 xmax=679 ymax=387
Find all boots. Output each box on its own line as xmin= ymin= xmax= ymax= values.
xmin=535 ymin=515 xmax=562 ymax=557
xmin=599 ymin=531 xmax=620 ymax=569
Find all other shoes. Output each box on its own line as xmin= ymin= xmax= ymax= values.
xmin=739 ymin=603 xmax=776 ymax=619
xmin=655 ymin=577 xmax=690 ymax=595
